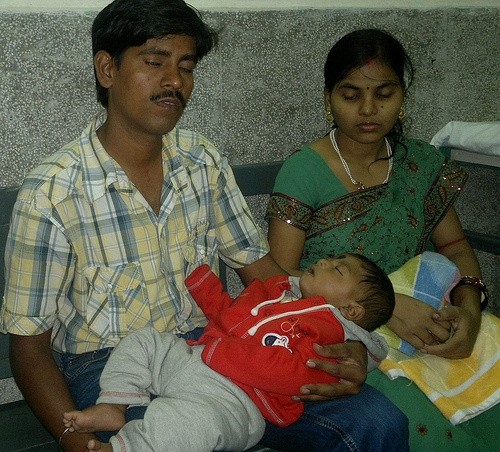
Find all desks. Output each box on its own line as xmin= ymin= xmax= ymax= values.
xmin=429 ymin=119 xmax=500 ymax=255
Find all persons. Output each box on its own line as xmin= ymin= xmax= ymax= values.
xmin=62 ymin=252 xmax=395 ymax=452
xmin=265 ymin=30 xmax=500 ymax=452
xmin=0 ymin=0 xmax=410 ymax=452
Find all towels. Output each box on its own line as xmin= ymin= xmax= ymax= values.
xmin=370 ymin=249 xmax=500 ymax=426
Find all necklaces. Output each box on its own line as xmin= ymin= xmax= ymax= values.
xmin=330 ymin=128 xmax=394 ymax=189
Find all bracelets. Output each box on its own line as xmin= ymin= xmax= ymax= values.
xmin=450 ymin=276 xmax=489 ymax=312
xmin=58 ymin=428 xmax=69 ymax=452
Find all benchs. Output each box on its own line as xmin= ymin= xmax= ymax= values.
xmin=0 ymin=158 xmax=500 ymax=452
